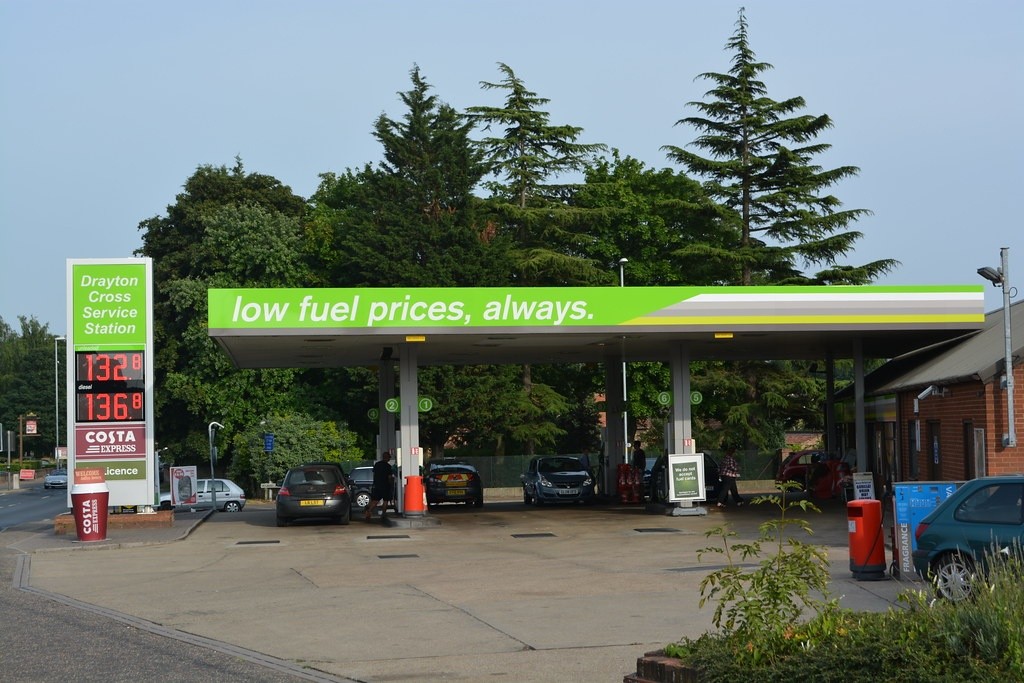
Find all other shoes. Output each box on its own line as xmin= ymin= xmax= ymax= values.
xmin=737 ymin=502 xmax=745 ymax=506
xmin=716 ymin=502 xmax=729 ymax=507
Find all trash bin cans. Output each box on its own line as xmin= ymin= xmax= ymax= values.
xmin=403 ymin=475 xmax=425 ymax=519
xmin=846 ymin=498 xmax=887 ymax=580
xmin=833 ymin=462 xmax=850 ymax=493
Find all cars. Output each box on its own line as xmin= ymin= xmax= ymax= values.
xmin=643 ymin=458 xmax=658 ymax=496
xmin=276 ymin=465 xmax=356 ymax=527
xmin=912 ymin=473 xmax=1024 ymax=604
xmin=704 ymin=453 xmax=719 ymax=498
xmin=646 ymin=457 xmax=661 ymax=501
xmin=348 ymin=466 xmax=395 ymax=507
xmin=421 ymin=459 xmax=483 ymax=508
xmin=44 ymin=469 xmax=67 ymax=489
xmin=523 ymin=455 xmax=592 ymax=505
xmin=155 ymin=479 xmax=246 ymax=513
xmin=300 ymin=462 xmax=351 ymax=492
xmin=42 ymin=460 xmax=50 ymax=464
xmin=775 ymin=449 xmax=826 ymax=492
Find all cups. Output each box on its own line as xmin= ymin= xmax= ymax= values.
xmin=69 ymin=483 xmax=110 ymax=541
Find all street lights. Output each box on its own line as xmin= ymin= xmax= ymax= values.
xmin=54 ymin=337 xmax=66 ymax=470
xmin=619 ymin=257 xmax=628 ymax=463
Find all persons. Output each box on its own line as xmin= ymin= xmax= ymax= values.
xmin=717 ymin=446 xmax=745 ymax=507
xmin=366 ymin=452 xmax=394 ymax=522
xmin=632 ymin=441 xmax=646 ymax=480
xmin=581 ymin=447 xmax=590 ymax=466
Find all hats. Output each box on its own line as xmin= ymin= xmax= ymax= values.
xmin=178 ymin=476 xmax=191 ymax=491
